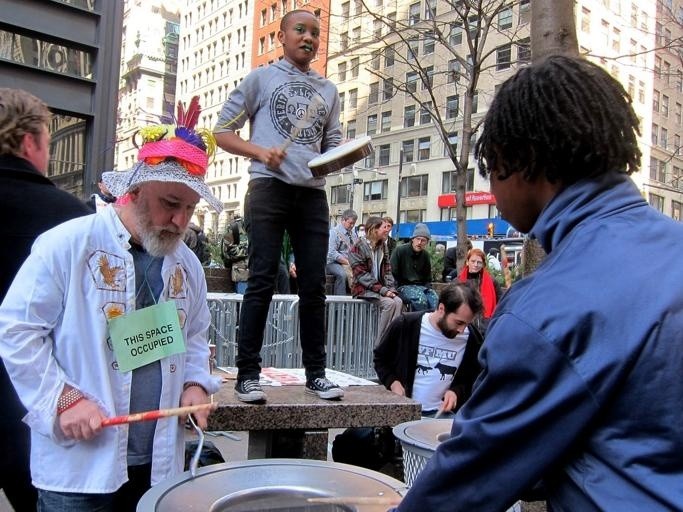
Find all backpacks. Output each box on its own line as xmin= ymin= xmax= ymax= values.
xmin=188 ymin=226 xmax=212 ymax=267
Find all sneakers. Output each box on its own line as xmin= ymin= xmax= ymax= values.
xmin=234 ymin=377 xmax=271 ymax=404
xmin=306 ymin=375 xmax=344 ymax=399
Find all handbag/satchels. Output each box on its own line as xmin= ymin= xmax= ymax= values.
xmin=220 ymin=221 xmax=246 ymax=269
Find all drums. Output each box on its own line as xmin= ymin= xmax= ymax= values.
xmin=308 ymin=135 xmax=373 ymax=176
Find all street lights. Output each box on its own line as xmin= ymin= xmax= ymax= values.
xmin=395 ymin=146 xmax=415 ymax=222
xmin=349 ymin=164 xmax=387 ymax=210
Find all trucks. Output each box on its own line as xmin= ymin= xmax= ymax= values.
xmin=427 ymin=233 xmax=524 ymax=276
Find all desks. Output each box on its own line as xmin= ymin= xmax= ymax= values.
xmin=175 ymin=363 xmax=424 ymax=464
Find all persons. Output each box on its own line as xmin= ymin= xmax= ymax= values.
xmin=333 ymin=281 xmax=486 ymax=470
xmin=0 ymin=96 xmax=222 ymax=510
xmin=212 ymin=9 xmax=343 ymax=402
xmin=390 ymin=54 xmax=681 ymax=511
xmin=1 ymin=88 xmax=97 ymax=512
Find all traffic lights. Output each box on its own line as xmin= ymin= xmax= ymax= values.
xmin=487 ymin=225 xmax=494 ymax=238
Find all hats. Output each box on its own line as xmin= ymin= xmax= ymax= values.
xmin=412 ymin=222 xmax=431 ymax=238
xmin=100 ymin=127 xmax=224 ymax=214
